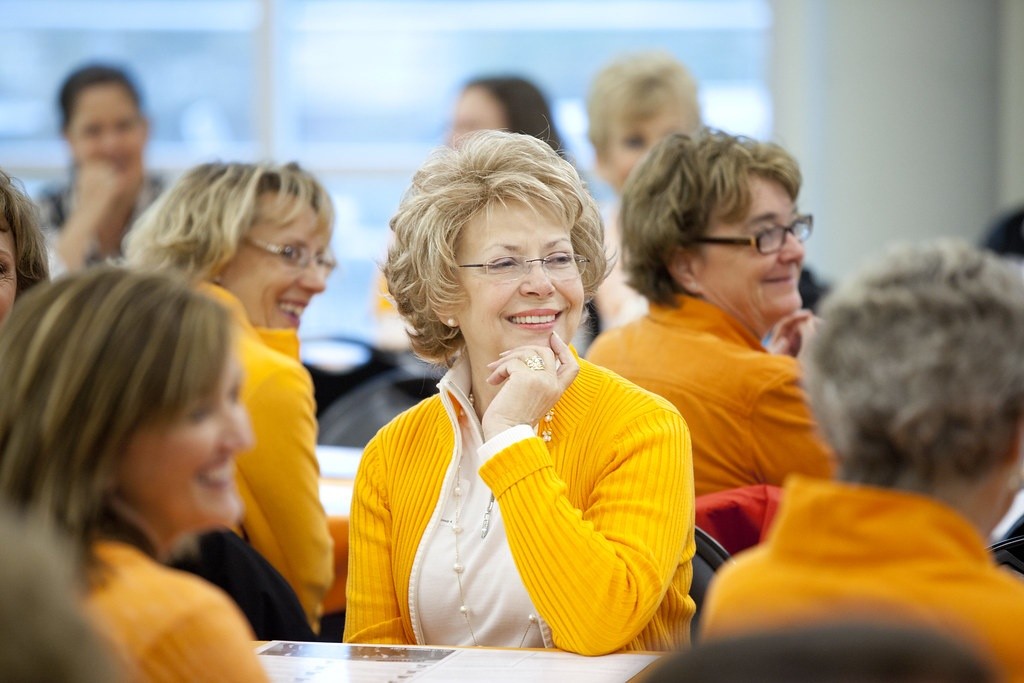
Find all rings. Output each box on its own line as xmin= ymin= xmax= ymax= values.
xmin=524 ymin=354 xmax=545 ymax=370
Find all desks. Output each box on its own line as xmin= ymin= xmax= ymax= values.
xmin=252 ymin=646 xmax=674 ymax=683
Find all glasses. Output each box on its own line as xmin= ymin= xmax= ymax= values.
xmin=458 ymin=248 xmax=590 ymax=280
xmin=697 ymin=214 xmax=813 ymax=255
xmin=243 ymin=233 xmax=336 ymax=274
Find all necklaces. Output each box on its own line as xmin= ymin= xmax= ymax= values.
xmin=451 ymin=393 xmax=555 ymax=647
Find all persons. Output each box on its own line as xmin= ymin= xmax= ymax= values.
xmin=113 ymin=154 xmax=337 ymax=640
xmin=585 ymin=122 xmax=841 ymax=505
xmin=575 ymin=47 xmax=708 ymax=332
xmin=32 ymin=60 xmax=171 ymax=280
xmin=341 ymin=129 xmax=697 ymax=657
xmin=693 ymin=232 xmax=1022 ymax=682
xmin=0 ymin=265 xmax=270 ymax=682
